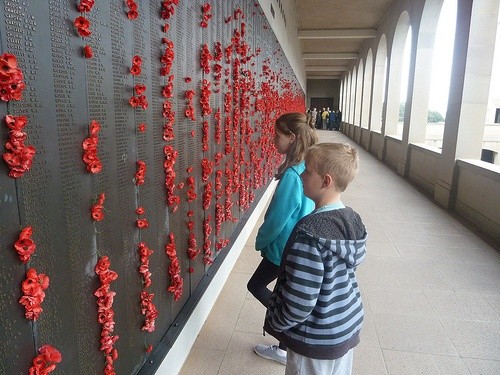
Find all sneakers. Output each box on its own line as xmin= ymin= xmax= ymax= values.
xmin=253 ymin=345 xmax=288 ymax=366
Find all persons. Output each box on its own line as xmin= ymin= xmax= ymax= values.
xmin=306 ymin=108 xmax=342 ymax=131
xmin=247 ymin=110 xmax=319 ymax=367
xmin=262 ymin=143 xmax=367 ymax=375
